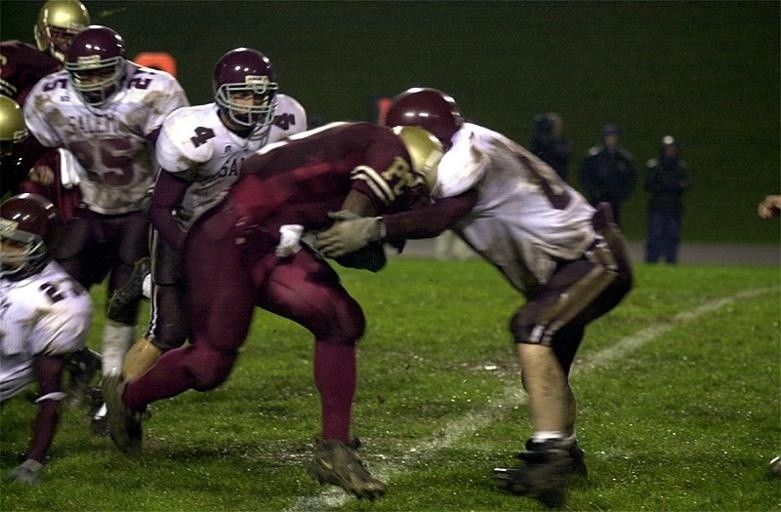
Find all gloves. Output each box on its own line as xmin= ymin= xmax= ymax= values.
xmin=314 ymin=208 xmax=383 ymax=260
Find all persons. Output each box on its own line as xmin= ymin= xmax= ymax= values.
xmin=758 ymin=195 xmax=780 ymax=478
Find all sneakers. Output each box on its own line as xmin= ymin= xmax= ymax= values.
xmin=308 ymin=435 xmax=388 ymax=501
xmin=106 ymin=256 xmax=152 ymax=321
xmin=492 ymin=436 xmax=588 ymax=496
xmin=67 ymin=345 xmax=153 ymax=460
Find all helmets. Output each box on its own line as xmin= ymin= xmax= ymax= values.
xmin=31 ymin=0 xmax=127 ymax=107
xmin=1 ymin=91 xmax=29 ymax=160
xmin=214 ymin=46 xmax=278 ymax=127
xmin=385 ymin=86 xmax=463 ymax=198
xmin=0 ymin=193 xmax=58 ymax=282
